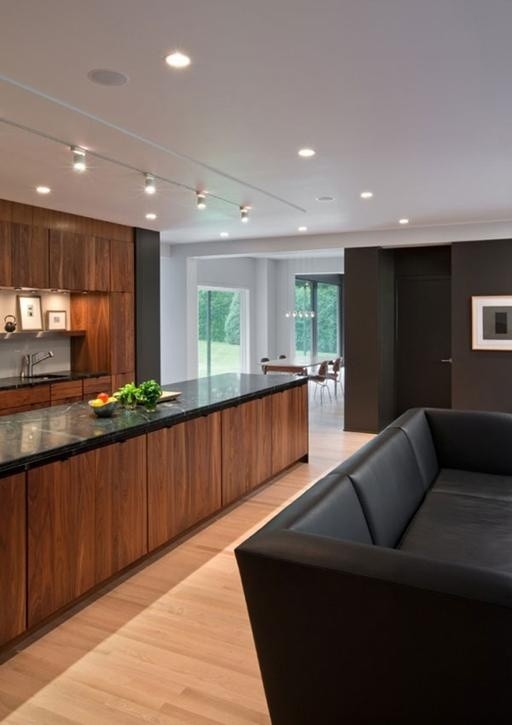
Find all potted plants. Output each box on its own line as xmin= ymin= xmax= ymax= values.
xmin=136 ymin=380 xmax=162 ymax=414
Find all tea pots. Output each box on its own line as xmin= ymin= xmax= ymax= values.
xmin=4 ymin=314 xmax=17 ymax=332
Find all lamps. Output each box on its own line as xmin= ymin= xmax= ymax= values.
xmin=0 ymin=116 xmax=252 ymax=223
xmin=284 ymin=285 xmax=315 ymax=318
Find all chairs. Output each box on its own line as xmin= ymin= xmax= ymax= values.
xmin=261 ymin=354 xmax=344 ymax=404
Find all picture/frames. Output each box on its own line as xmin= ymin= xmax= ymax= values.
xmin=46 ymin=310 xmax=66 ymax=331
xmin=16 ymin=295 xmax=43 ymax=331
xmin=472 ymin=296 xmax=512 ymax=351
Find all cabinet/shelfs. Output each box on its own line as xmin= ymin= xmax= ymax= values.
xmin=0 ymin=373 xmax=136 ymax=417
xmin=0 ymin=198 xmax=134 ymax=292
xmin=70 ymin=293 xmax=135 ymax=373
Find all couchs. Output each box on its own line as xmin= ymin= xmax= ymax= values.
xmin=233 ymin=405 xmax=512 ymax=725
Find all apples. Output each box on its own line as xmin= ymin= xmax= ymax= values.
xmin=98 ymin=392 xmax=109 ymax=402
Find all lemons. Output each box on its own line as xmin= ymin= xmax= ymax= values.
xmin=92 ymin=399 xmax=102 ymax=407
xmin=106 ymin=397 xmax=117 ymax=404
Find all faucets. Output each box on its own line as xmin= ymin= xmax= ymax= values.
xmin=27 ymin=350 xmax=54 ymax=376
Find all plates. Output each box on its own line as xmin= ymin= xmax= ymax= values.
xmin=141 ymin=390 xmax=182 ymax=405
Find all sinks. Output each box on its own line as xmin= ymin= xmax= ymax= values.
xmin=33 ymin=373 xmax=67 ymax=378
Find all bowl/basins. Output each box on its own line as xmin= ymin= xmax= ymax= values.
xmin=88 ymin=399 xmax=119 ymax=417
